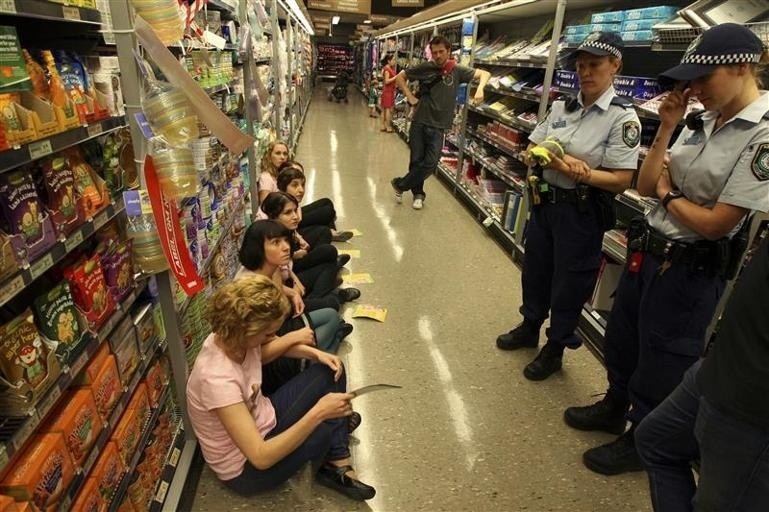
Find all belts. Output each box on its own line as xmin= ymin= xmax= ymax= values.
xmin=547 ymin=186 xmax=597 ymax=205
xmin=641 ymin=225 xmax=693 ymax=266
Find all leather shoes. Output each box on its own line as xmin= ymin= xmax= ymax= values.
xmin=316 ymin=226 xmax=376 ymax=500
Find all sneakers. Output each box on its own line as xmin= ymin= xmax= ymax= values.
xmin=412 ymin=199 xmax=422 ymax=209
xmin=391 ymin=179 xmax=402 ymax=203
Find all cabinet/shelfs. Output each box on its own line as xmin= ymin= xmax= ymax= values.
xmin=0 ymin=0 xmax=316 ymax=512
xmin=354 ymin=0 xmax=769 ymax=370
xmin=318 ymin=46 xmax=353 ymax=82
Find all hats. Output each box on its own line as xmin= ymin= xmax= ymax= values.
xmin=657 ymin=23 xmax=764 ymax=81
xmin=568 ymin=31 xmax=626 ymax=58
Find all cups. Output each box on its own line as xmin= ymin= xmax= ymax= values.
xmin=124 ymin=1 xmax=203 ymax=273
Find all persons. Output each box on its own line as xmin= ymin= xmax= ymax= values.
xmin=234 ymin=220 xmax=353 ymax=397
xmin=257 ymin=141 xmax=353 ymax=242
xmin=390 ymin=36 xmax=491 ymax=210
xmin=564 ymin=23 xmax=769 ymax=474
xmin=187 ymin=274 xmax=376 ymax=501
xmin=260 ymin=191 xmax=361 ymax=311
xmin=634 ymin=243 xmax=769 ymax=512
xmin=496 ymin=31 xmax=643 ymax=380
xmin=380 ymin=54 xmax=404 ymax=133
xmin=254 ymin=167 xmax=351 ymax=271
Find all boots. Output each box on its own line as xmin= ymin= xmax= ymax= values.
xmin=564 ymin=381 xmax=629 ymax=434
xmin=497 ymin=315 xmax=544 ymax=348
xmin=524 ymin=339 xmax=565 ymax=380
xmin=583 ymin=421 xmax=647 ymax=475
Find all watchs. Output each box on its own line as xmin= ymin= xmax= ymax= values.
xmin=663 ymin=190 xmax=685 ymax=206
